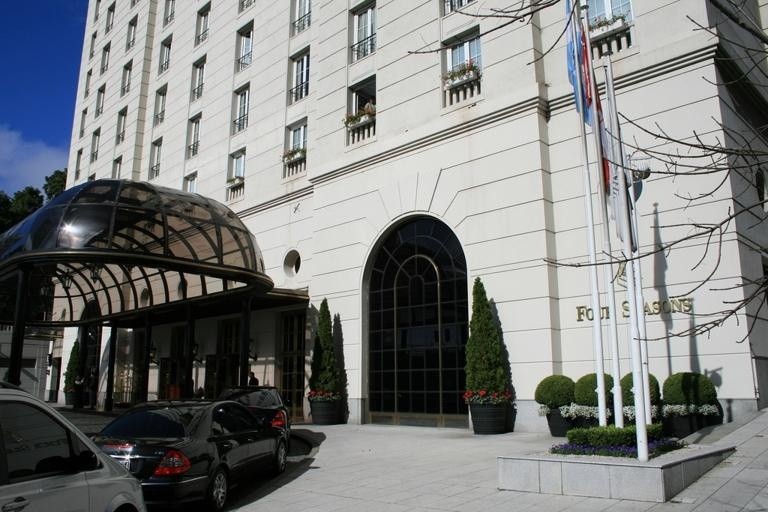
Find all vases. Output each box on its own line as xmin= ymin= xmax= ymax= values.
xmin=469 ymin=402 xmax=509 ymax=434
xmin=309 ymin=400 xmax=345 ymax=425
xmin=544 ymin=410 xmax=714 ymax=439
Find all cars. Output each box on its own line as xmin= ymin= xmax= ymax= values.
xmin=0 ymin=381 xmax=292 ymax=512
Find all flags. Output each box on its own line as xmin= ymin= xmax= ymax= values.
xmin=566 ymin=0 xmax=638 ymax=252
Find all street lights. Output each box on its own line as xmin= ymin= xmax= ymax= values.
xmin=627 ymin=154 xmax=652 ymax=424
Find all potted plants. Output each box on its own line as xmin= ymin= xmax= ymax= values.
xmin=62 ymin=338 xmax=80 ymax=406
xmin=587 ymin=7 xmax=632 ymax=39
xmin=341 ymin=105 xmax=373 ymax=129
xmin=441 ymin=58 xmax=481 ymax=88
xmin=225 ymin=176 xmax=244 ymax=190
xmin=281 ymin=146 xmax=306 ymax=164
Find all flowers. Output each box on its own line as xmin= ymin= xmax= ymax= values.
xmin=307 ymin=297 xmax=344 ymax=403
xmin=534 ymin=370 xmax=723 ymax=421
xmin=462 ymin=276 xmax=516 ymax=404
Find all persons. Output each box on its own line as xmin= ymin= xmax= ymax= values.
xmin=249 ymin=372 xmax=258 ymax=386
xmin=364 ymin=95 xmax=376 ymax=112
xmin=73 ymin=372 xmax=97 ymax=409
